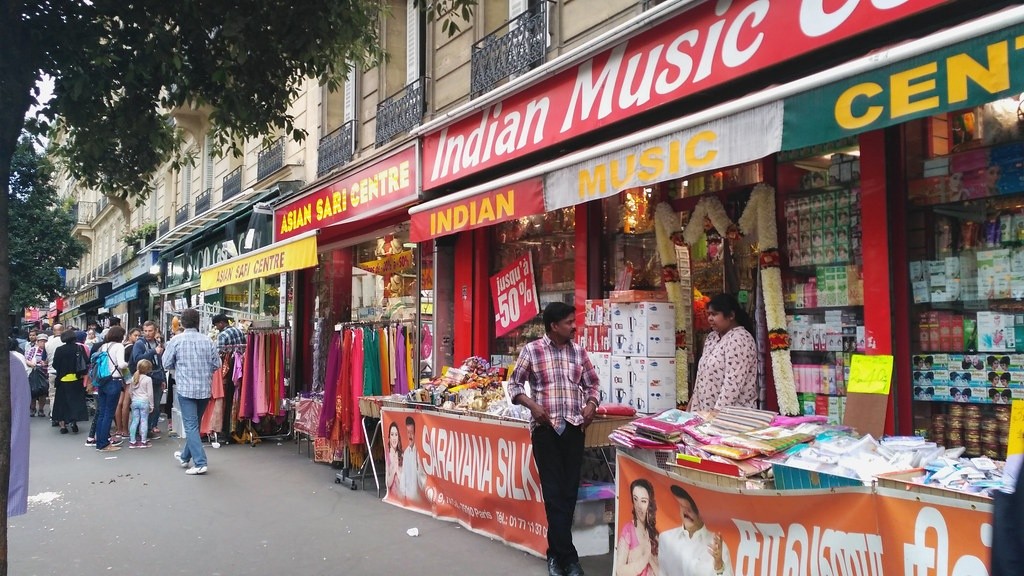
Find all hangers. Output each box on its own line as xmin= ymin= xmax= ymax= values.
xmin=247 ymin=326 xmax=283 ymax=336
xmin=337 ymin=316 xmax=413 ymax=345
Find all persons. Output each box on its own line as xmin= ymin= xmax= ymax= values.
xmin=946 ymin=171 xmax=970 ymax=202
xmin=7 ymin=313 xmax=185 ymax=454
xmin=788 ymin=331 xmax=811 ymax=348
xmin=814 ymin=332 xmax=843 ymax=346
xmin=210 ymin=312 xmax=247 ymax=446
xmin=656 ymin=485 xmax=734 ymax=576
xmin=859 ymin=339 xmax=865 ymax=349
xmin=161 ymin=308 xmax=223 ymax=476
xmin=504 ymin=299 xmax=604 ymax=576
xmin=782 ymin=168 xmax=863 ymax=267
xmin=385 ymin=416 xmax=429 ymax=502
xmin=684 ymin=293 xmax=761 ymax=414
xmin=978 ymin=161 xmax=1004 ymax=196
xmin=913 ymin=328 xmax=1012 ymax=405
xmin=615 ymin=478 xmax=660 ymax=576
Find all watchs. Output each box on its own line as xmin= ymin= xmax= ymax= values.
xmin=588 ymin=400 xmax=599 ymax=413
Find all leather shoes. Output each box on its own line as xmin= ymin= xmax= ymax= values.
xmin=547 ymin=556 xmax=566 ymax=576
xmin=568 ymin=560 xmax=584 ymax=576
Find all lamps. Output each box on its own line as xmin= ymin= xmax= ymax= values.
xmin=621 ymin=186 xmax=649 ymax=234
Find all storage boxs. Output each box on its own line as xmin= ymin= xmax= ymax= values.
xmin=589 ymin=290 xmax=676 ymax=413
xmin=786 ymin=152 xmax=866 ymax=427
xmin=908 ymin=134 xmax=1024 ymax=457
xmin=626 ymin=447 xmax=997 ymax=504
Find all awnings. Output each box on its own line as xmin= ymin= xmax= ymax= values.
xmin=103 ymin=281 xmax=140 ymax=309
xmin=199 ymin=228 xmax=321 ymax=292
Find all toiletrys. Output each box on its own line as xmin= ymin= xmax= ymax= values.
xmin=812 ymin=323 xmax=826 ymax=350
xmin=932 ymin=214 xmax=1024 ymax=260
xmin=792 ymin=363 xmax=837 ymax=395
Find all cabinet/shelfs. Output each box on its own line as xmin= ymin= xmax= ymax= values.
xmin=910 ymin=190 xmax=1023 ymax=406
xmin=784 ymin=175 xmax=862 ymax=397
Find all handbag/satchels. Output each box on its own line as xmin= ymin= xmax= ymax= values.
xmin=28 ymin=367 xmax=50 ymax=397
xmin=145 ymin=365 xmax=166 ymax=384
xmin=75 ymin=344 xmax=88 ymax=375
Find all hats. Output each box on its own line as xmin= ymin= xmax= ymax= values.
xmin=35 ymin=334 xmax=49 ymax=341
xmin=171 ymin=315 xmax=180 ymax=331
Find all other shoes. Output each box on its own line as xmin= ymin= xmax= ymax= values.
xmin=38 ymin=410 xmax=45 ymax=417
xmin=60 ymin=428 xmax=69 ymax=434
xmin=30 ymin=408 xmax=37 ymax=417
xmin=128 ymin=441 xmax=139 ymax=449
xmin=115 ymin=431 xmax=130 ymax=439
xmin=96 ymin=443 xmax=121 ymax=451
xmin=69 ymin=423 xmax=79 ymax=433
xmin=141 ymin=441 xmax=152 ymax=448
xmin=148 ymin=432 xmax=162 ymax=440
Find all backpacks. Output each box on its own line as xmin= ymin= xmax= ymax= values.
xmin=89 ymin=347 xmax=110 ymax=388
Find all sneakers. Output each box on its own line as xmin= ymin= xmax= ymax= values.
xmin=185 ymin=466 xmax=207 ymax=475
xmin=85 ymin=440 xmax=97 ymax=446
xmin=173 ymin=451 xmax=188 ymax=468
xmin=110 ymin=438 xmax=123 ymax=446
